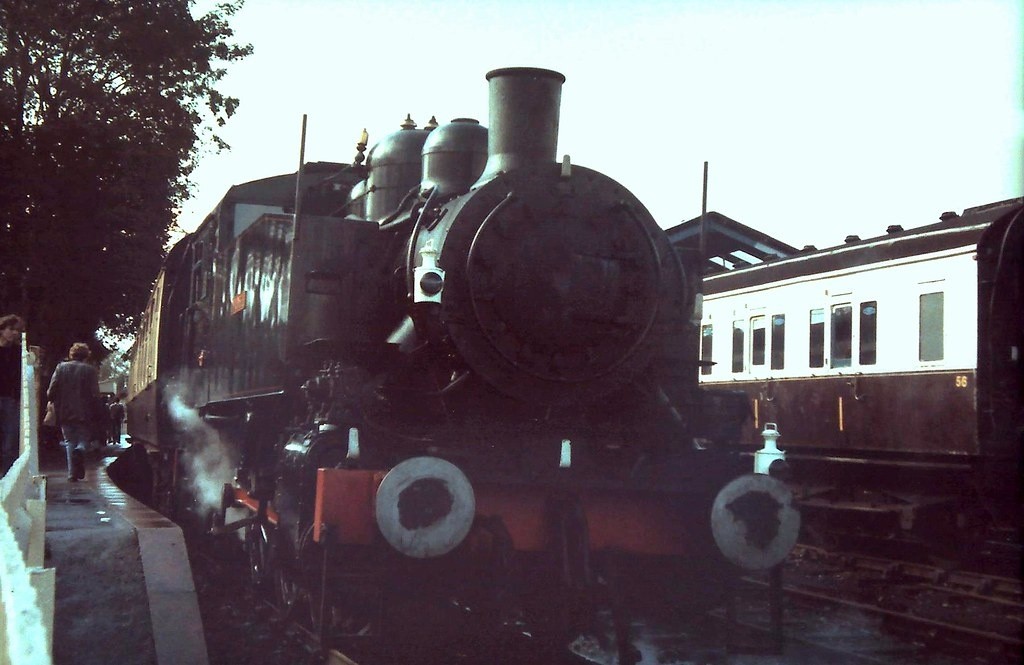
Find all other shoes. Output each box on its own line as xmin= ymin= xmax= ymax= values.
xmin=67 ymin=475 xmax=76 ymax=482
xmin=72 ymin=448 xmax=86 ymax=479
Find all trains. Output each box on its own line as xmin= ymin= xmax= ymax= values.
xmin=126 ymin=64 xmax=805 ymax=663
xmin=691 ymin=195 xmax=1023 ymax=520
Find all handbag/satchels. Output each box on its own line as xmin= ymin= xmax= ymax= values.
xmin=43 ymin=407 xmax=56 ymax=426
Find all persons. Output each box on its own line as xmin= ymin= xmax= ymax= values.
xmin=95 ymin=394 xmax=125 ymax=446
xmin=41 ymin=343 xmax=100 ymax=482
xmin=0 ymin=313 xmax=26 ymax=479
